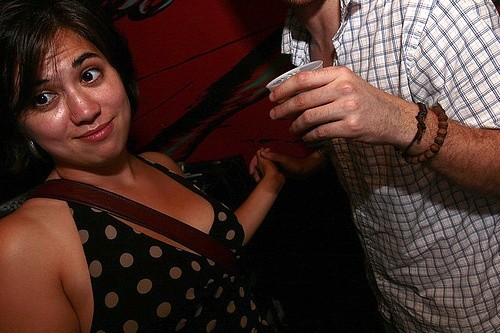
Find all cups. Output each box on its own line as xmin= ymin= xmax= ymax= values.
xmin=265 ymin=59 xmax=337 ymax=146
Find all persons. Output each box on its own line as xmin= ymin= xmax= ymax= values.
xmin=249 ymin=0 xmax=500 ymax=333
xmin=1 ymin=2 xmax=290 ymax=333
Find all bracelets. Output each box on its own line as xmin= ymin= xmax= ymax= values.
xmin=399 ymin=103 xmax=424 ymax=150
xmin=400 ymin=101 xmax=449 ymax=165
